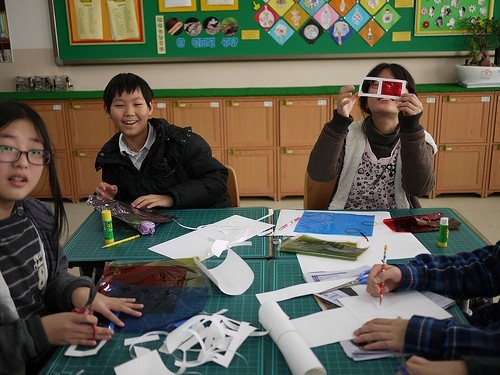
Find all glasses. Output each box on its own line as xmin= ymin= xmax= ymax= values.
xmin=0 ymin=146 xmax=53 ymax=166
xmin=370 ymin=80 xmax=379 ymax=89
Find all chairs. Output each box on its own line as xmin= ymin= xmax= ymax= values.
xmin=223 ymin=164 xmax=239 ymax=207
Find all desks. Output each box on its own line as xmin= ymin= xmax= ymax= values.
xmin=38 ymin=202 xmax=497 ymax=375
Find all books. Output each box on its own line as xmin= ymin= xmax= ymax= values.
xmin=304 ymin=263 xmax=456 ymax=362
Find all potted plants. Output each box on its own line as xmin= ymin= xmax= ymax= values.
xmin=454 ymin=12 xmax=500 ymax=86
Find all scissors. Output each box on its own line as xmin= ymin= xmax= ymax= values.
xmin=72 ymin=268 xmax=105 ymax=341
xmin=318 ymin=268 xmax=372 ymax=295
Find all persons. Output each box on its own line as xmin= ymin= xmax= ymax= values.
xmin=307 ymin=62 xmax=438 ymax=211
xmin=405 ymin=354 xmax=500 ymax=375
xmin=94 ymin=73 xmax=233 ymax=209
xmin=352 ymin=238 xmax=500 ymax=359
xmin=0 ymin=100 xmax=144 ymax=375
xmin=435 ymin=18 xmax=442 ymax=27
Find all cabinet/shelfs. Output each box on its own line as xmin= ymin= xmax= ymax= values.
xmin=23 ymin=92 xmax=500 ymax=204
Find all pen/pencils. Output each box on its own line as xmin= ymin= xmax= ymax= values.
xmin=379 ymin=244 xmax=388 ymax=306
xmin=101 ymin=234 xmax=140 ymax=248
xmin=279 ymin=217 xmax=301 ymax=231
